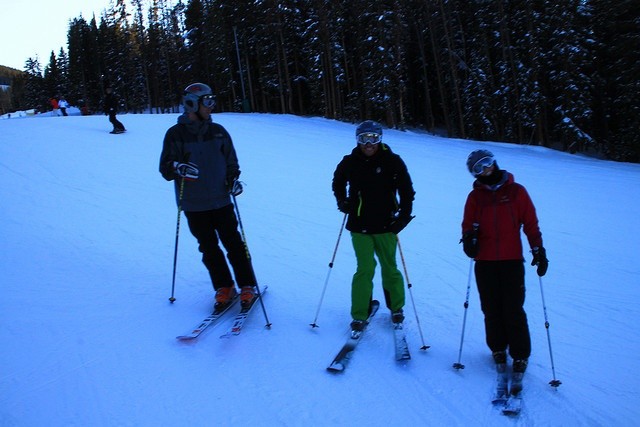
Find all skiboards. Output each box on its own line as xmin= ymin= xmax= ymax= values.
xmin=327 ymin=300 xmax=410 ymax=375
xmin=176 ymin=284 xmax=268 ymax=340
xmin=492 ymin=372 xmax=522 ymax=415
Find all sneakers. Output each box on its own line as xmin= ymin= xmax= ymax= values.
xmin=240 ymin=286 xmax=254 ymax=307
xmin=389 ymin=308 xmax=405 ymax=323
xmin=492 ymin=350 xmax=508 ymax=363
xmin=351 ymin=318 xmax=367 ymax=330
xmin=214 ymin=279 xmax=237 ymax=304
xmin=513 ymin=357 xmax=528 ymax=372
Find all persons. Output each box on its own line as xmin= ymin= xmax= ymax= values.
xmin=461 ymin=148 xmax=548 ymax=373
xmin=159 ymin=82 xmax=255 ymax=310
xmin=58 ymin=95 xmax=68 ymax=117
xmin=49 ymin=97 xmax=59 ymax=116
xmin=331 ymin=120 xmax=416 ymax=331
xmin=100 ymin=83 xmax=127 ymax=134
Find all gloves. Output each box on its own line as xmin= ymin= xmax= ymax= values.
xmin=226 ymin=169 xmax=243 ymax=196
xmin=170 ymin=159 xmax=200 ymax=182
xmin=460 ymin=233 xmax=481 ymax=258
xmin=531 ymin=248 xmax=549 ymax=277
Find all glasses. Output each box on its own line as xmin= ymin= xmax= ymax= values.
xmin=195 ymin=94 xmax=218 ymax=108
xmin=356 ymin=132 xmax=382 ymax=146
xmin=471 ymin=156 xmax=495 ymax=178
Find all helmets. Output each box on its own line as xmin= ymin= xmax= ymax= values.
xmin=355 ymin=120 xmax=383 ymax=147
xmin=182 ymin=82 xmax=214 ymax=114
xmin=466 ymin=149 xmax=496 ymax=176
